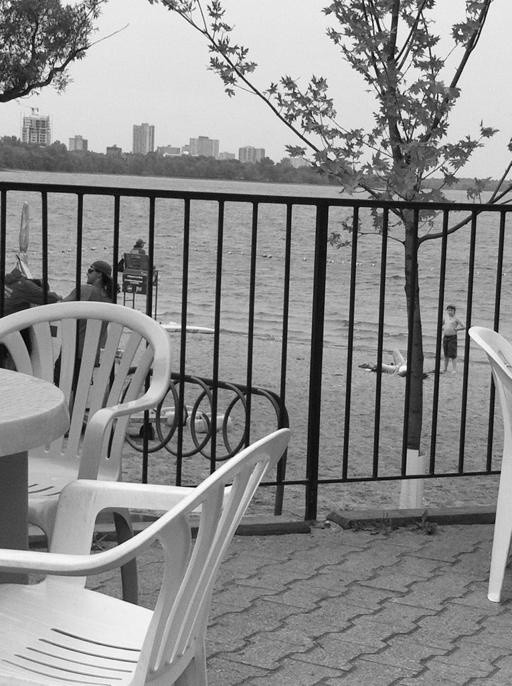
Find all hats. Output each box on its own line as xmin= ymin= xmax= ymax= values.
xmin=136 ymin=239 xmax=146 ymax=245
xmin=92 ymin=261 xmax=113 ymax=280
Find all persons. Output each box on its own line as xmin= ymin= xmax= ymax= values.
xmin=0 ymin=278 xmax=61 ymax=369
xmin=130 ymin=239 xmax=145 ymax=254
xmin=54 ymin=261 xmax=114 ymax=438
xmin=440 ymin=306 xmax=471 ymax=373
xmin=361 ymin=350 xmax=430 ymax=381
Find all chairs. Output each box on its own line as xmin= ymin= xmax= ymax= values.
xmin=1 ymin=428 xmax=292 ymax=686
xmin=467 ymin=325 xmax=512 ymax=604
xmin=1 ymin=299 xmax=174 ymax=604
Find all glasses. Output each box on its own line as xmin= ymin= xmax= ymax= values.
xmin=87 ymin=269 xmax=96 ymax=273
